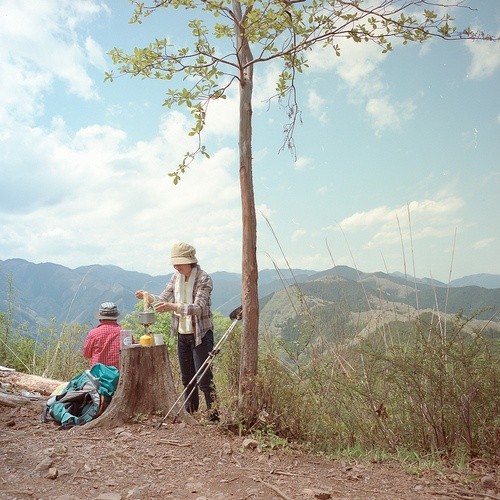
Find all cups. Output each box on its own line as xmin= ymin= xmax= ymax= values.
xmin=120 ymin=330 xmax=132 ymax=350
xmin=152 ymin=301 xmax=159 ymax=310
xmin=154 ymin=334 xmax=164 ymax=345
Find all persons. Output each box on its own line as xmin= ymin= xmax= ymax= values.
xmin=82 ymin=300 xmax=137 ymax=372
xmin=135 ymin=241 xmax=222 ymax=423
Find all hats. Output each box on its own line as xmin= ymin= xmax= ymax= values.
xmin=94 ymin=302 xmax=122 ymax=321
xmin=168 ymin=242 xmax=198 ymax=266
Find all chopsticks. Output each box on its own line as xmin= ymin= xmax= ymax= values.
xmin=140 ymin=291 xmax=148 ymax=294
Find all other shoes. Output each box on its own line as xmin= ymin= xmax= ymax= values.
xmin=204 ymin=411 xmax=219 ymax=422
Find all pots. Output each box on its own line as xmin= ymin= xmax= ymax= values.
xmin=139 ymin=312 xmax=155 ymax=323
